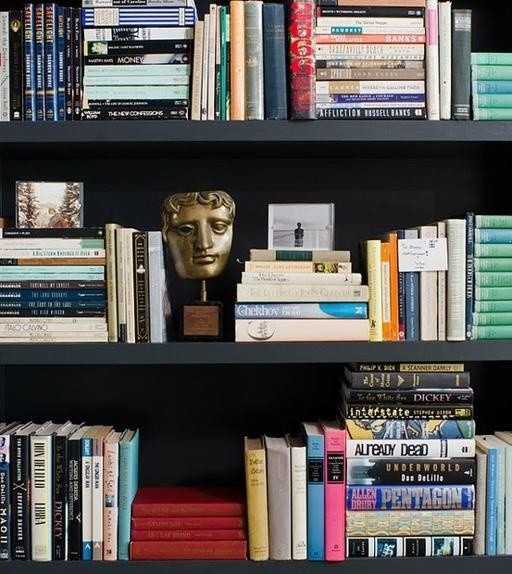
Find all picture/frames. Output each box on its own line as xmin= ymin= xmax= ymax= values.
xmin=265 ymin=201 xmax=335 ymax=249
xmin=13 ymin=180 xmax=85 ymax=229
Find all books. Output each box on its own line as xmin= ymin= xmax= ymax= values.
xmin=0 ymin=174 xmax=167 ymax=348
xmin=234 ymin=204 xmax=512 ymax=342
xmin=1 ymin=421 xmax=248 ymax=560
xmin=244 ymin=364 xmax=512 ymax=560
xmin=0 ymin=0 xmax=512 ymax=122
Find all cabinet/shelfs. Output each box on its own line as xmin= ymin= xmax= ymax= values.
xmin=0 ymin=120 xmax=512 ymax=574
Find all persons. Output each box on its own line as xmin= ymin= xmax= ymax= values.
xmin=163 ymin=189 xmax=238 ymax=278
